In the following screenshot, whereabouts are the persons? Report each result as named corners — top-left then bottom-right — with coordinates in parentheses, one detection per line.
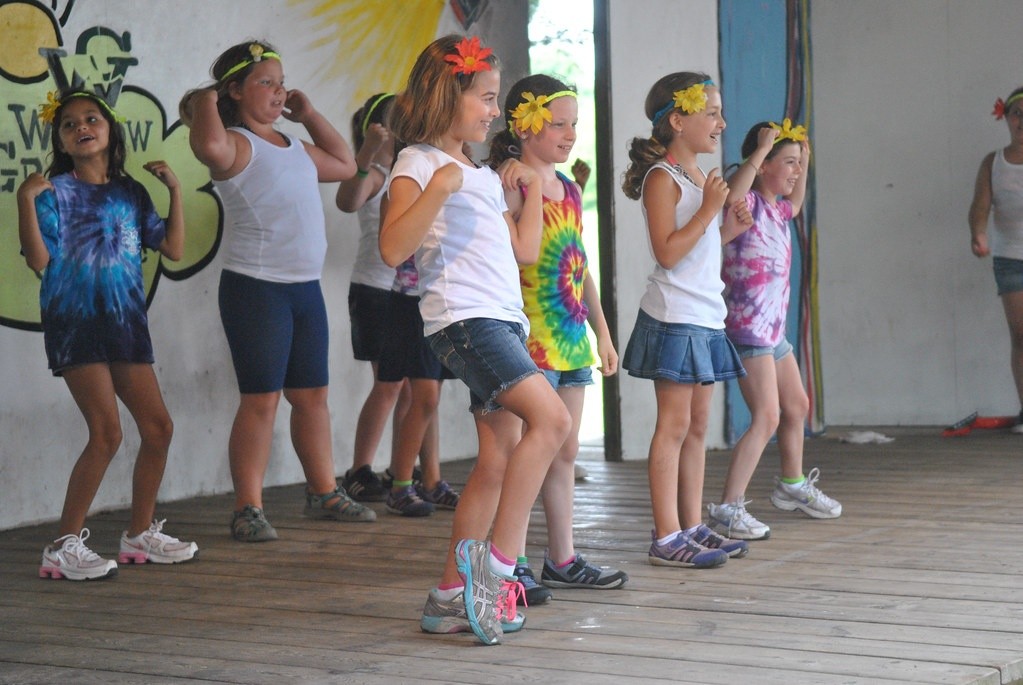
top-left (489, 73), bottom-right (631, 605)
top-left (17, 90), bottom-right (197, 579)
top-left (623, 71), bottom-right (747, 568)
top-left (335, 91), bottom-right (460, 518)
top-left (179, 41), bottom-right (377, 541)
top-left (969, 88), bottom-right (1023, 432)
top-left (707, 118), bottom-right (842, 540)
top-left (380, 34), bottom-right (571, 644)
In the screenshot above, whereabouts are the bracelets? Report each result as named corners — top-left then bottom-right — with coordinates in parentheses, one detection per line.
top-left (749, 161), bottom-right (759, 175)
top-left (356, 171), bottom-right (368, 178)
top-left (693, 214), bottom-right (706, 233)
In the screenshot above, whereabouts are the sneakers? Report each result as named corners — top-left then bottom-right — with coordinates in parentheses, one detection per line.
top-left (381, 468), bottom-right (424, 492)
top-left (540, 548), bottom-right (628, 589)
top-left (422, 481), bottom-right (459, 509)
top-left (343, 465), bottom-right (387, 502)
top-left (688, 525), bottom-right (749, 558)
top-left (648, 528), bottom-right (730, 567)
top-left (455, 538), bottom-right (527, 645)
top-left (39, 528), bottom-right (117, 581)
top-left (512, 565), bottom-right (552, 604)
top-left (118, 518), bottom-right (198, 564)
top-left (420, 587), bottom-right (526, 634)
top-left (706, 497), bottom-right (770, 540)
top-left (231, 505), bottom-right (277, 540)
top-left (304, 486), bottom-right (375, 521)
top-left (769, 467), bottom-right (842, 519)
top-left (384, 487), bottom-right (430, 517)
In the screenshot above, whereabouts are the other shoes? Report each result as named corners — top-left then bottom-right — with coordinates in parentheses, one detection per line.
top-left (574, 463), bottom-right (588, 477)
top-left (1008, 410), bottom-right (1023, 434)
